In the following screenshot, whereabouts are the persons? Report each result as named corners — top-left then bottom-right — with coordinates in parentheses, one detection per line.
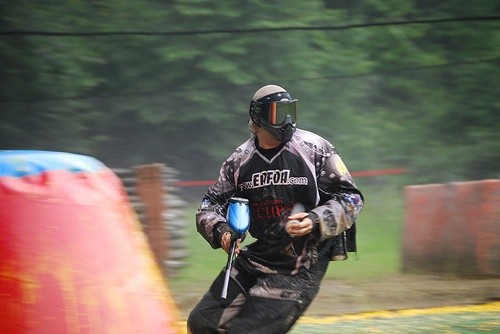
top-left (186, 82), bottom-right (366, 333)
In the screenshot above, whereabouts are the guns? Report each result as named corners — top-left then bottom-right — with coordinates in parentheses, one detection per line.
top-left (217, 196), bottom-right (251, 300)
